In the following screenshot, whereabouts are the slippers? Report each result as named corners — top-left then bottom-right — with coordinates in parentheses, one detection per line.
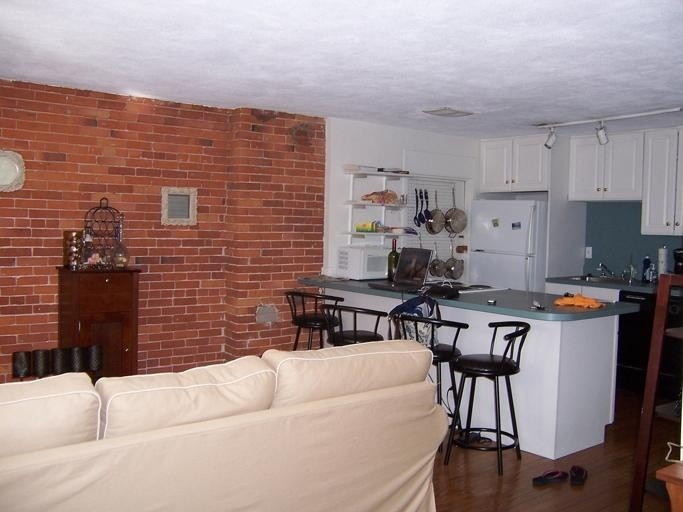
top-left (569, 465), bottom-right (587, 485)
top-left (533, 469), bottom-right (569, 487)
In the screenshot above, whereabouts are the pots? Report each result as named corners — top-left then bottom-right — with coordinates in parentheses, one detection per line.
top-left (429, 241), bottom-right (446, 277)
top-left (425, 189), bottom-right (445, 235)
top-left (444, 186), bottom-right (467, 238)
top-left (444, 240), bottom-right (463, 280)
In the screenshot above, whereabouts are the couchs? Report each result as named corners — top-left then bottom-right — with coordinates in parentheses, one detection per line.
top-left (0, 339), bottom-right (451, 512)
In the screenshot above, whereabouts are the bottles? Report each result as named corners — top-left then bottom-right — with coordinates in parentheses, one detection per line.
top-left (388, 239), bottom-right (399, 281)
top-left (656, 246), bottom-right (669, 281)
top-left (641, 255), bottom-right (650, 284)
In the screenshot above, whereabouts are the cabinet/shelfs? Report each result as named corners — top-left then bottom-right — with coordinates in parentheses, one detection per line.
top-left (480, 135), bottom-right (551, 197)
top-left (52, 266), bottom-right (142, 385)
top-left (340, 166), bottom-right (412, 246)
top-left (627, 273), bottom-right (681, 512)
top-left (638, 129), bottom-right (682, 237)
top-left (569, 133), bottom-right (644, 205)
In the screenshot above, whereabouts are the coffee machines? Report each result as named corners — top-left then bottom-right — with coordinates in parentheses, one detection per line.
top-left (672, 248), bottom-right (683, 274)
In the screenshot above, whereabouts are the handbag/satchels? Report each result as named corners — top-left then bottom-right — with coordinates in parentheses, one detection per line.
top-left (388, 297), bottom-right (440, 347)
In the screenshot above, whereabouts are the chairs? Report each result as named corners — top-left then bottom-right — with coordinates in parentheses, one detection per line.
top-left (320, 302), bottom-right (388, 346)
top-left (386, 309), bottom-right (469, 450)
top-left (442, 321), bottom-right (531, 476)
top-left (283, 290), bottom-right (344, 351)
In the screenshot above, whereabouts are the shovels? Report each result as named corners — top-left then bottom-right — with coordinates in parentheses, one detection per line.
top-left (424, 189), bottom-right (435, 223)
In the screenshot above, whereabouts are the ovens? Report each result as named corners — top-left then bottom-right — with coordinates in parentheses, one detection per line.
top-left (618, 290), bottom-right (682, 379)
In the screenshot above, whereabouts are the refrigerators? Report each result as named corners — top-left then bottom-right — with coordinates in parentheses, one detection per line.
top-left (466, 200), bottom-right (547, 294)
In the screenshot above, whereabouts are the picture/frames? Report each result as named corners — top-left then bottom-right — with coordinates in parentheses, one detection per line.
top-left (160, 187), bottom-right (198, 225)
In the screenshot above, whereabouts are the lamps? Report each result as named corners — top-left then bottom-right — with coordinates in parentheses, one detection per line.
top-left (545, 128), bottom-right (559, 150)
top-left (594, 121), bottom-right (609, 146)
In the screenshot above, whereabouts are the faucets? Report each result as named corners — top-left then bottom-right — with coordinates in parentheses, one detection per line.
top-left (596, 262), bottom-right (614, 278)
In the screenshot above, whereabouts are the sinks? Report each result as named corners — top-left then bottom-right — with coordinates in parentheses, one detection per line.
top-left (559, 275), bottom-right (608, 283)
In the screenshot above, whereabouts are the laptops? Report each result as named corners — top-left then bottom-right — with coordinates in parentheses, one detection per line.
top-left (368, 246), bottom-right (434, 292)
